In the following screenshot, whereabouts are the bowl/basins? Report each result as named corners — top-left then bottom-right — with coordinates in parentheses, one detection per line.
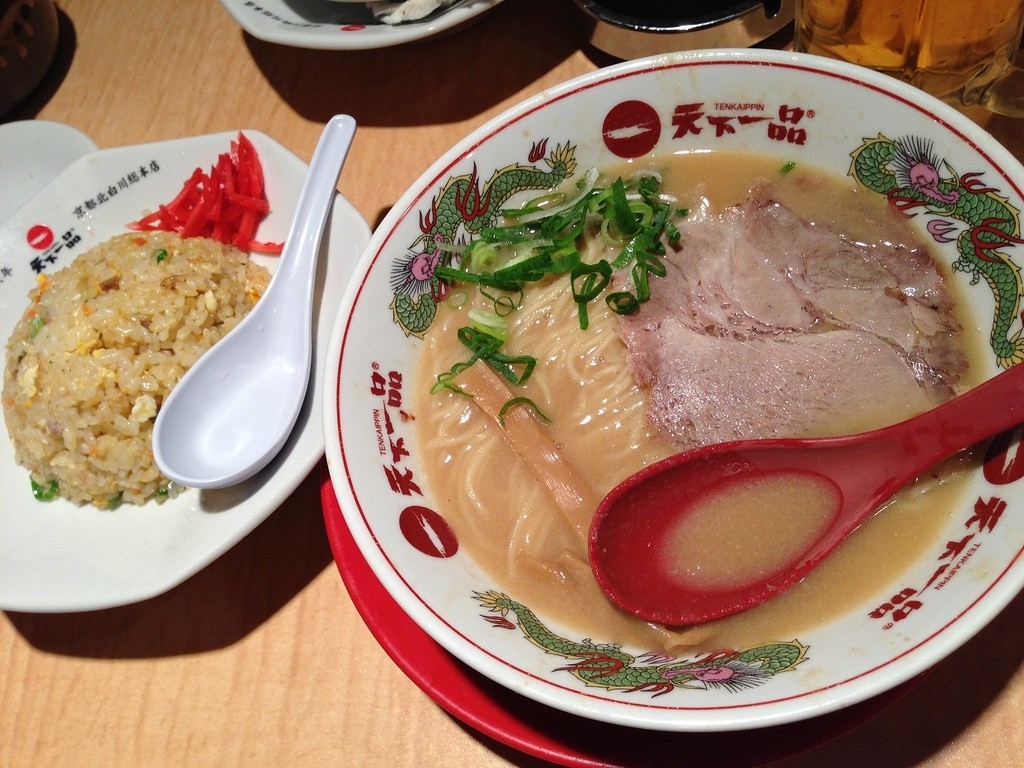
top-left (321, 48), bottom-right (1023, 735)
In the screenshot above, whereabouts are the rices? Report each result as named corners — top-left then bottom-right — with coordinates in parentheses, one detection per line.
top-left (0, 232), bottom-right (278, 508)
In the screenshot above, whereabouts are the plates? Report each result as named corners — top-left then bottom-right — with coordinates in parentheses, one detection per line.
top-left (575, 1), bottom-right (782, 34)
top-left (315, 462), bottom-right (986, 768)
top-left (1, 130), bottom-right (373, 615)
top-left (222, 1), bottom-right (504, 50)
top-left (2, 120), bottom-right (103, 228)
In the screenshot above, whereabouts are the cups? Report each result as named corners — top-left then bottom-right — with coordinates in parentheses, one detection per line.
top-left (1, 0), bottom-right (60, 121)
top-left (793, 1), bottom-right (1024, 120)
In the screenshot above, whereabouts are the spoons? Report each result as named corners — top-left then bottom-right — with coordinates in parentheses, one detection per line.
top-left (589, 357), bottom-right (1024, 627)
top-left (153, 112), bottom-right (359, 488)
